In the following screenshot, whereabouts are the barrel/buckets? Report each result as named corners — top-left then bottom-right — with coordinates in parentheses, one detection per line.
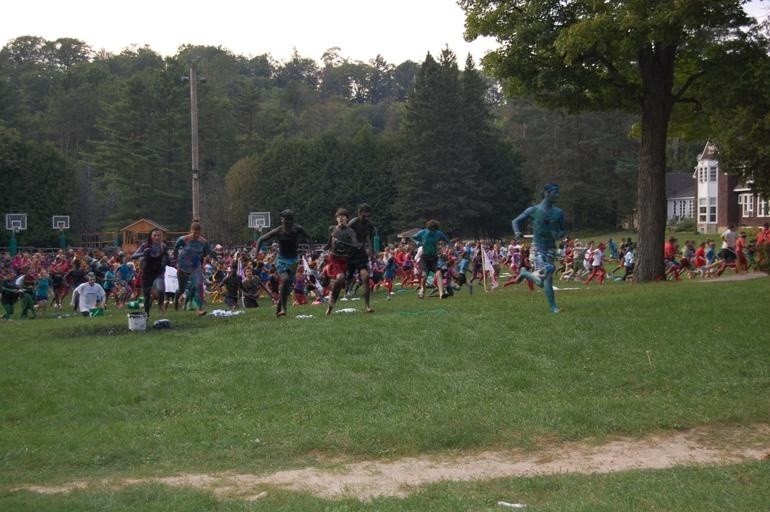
top-left (89, 308), bottom-right (104, 317)
top-left (125, 300), bottom-right (140, 310)
top-left (126, 310), bottom-right (149, 332)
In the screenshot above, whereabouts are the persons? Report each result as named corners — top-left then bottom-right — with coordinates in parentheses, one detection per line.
top-left (505, 183), bottom-right (565, 313)
top-left (254, 208), bottom-right (311, 316)
top-left (410, 219), bottom-right (452, 300)
top-left (346, 203), bottom-right (376, 314)
top-left (322, 208), bottom-right (362, 315)
top-left (0, 225), bottom-right (763, 322)
top-left (174, 222), bottom-right (212, 316)
